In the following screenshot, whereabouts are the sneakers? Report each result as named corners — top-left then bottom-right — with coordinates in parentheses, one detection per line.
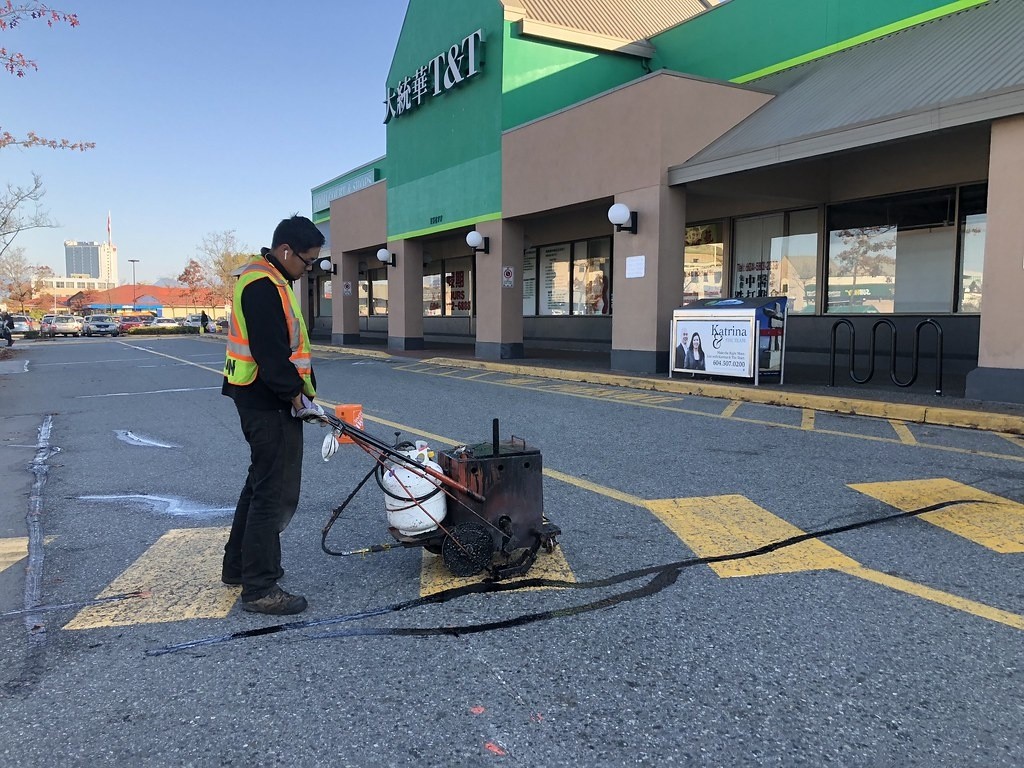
top-left (243, 587), bottom-right (307, 615)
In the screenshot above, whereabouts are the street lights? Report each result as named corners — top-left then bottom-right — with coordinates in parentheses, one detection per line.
top-left (129, 260), bottom-right (140, 311)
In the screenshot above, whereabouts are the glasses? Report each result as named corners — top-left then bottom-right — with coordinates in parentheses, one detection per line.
top-left (290, 245), bottom-right (319, 268)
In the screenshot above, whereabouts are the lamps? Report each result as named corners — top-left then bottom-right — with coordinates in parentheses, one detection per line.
top-left (320, 260), bottom-right (337, 275)
top-left (466, 231), bottom-right (489, 255)
top-left (608, 203), bottom-right (637, 234)
top-left (377, 249), bottom-right (396, 267)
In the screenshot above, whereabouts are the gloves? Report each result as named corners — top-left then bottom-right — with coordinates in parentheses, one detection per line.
top-left (291, 392), bottom-right (327, 427)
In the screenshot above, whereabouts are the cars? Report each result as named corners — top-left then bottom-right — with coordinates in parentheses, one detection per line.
top-left (47, 315), bottom-right (80, 338)
top-left (85, 314), bottom-right (118, 337)
top-left (216, 316), bottom-right (229, 333)
top-left (9, 312), bottom-right (186, 334)
top-left (182, 313), bottom-right (216, 333)
top-left (149, 317), bottom-right (179, 329)
top-left (116, 315), bottom-right (145, 337)
top-left (8, 316), bottom-right (33, 338)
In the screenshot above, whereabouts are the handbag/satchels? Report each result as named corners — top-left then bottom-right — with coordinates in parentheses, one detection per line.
top-left (200, 325), bottom-right (204, 334)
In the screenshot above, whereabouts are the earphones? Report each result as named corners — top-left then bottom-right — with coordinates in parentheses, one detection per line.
top-left (284, 250), bottom-right (288, 260)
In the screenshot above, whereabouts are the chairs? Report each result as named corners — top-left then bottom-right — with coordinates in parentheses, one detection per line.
top-left (67, 318), bottom-right (71, 322)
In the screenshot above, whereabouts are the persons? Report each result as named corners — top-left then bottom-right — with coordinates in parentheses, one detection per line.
top-left (2, 310), bottom-right (15, 347)
top-left (676, 328), bottom-right (705, 370)
top-left (201, 311), bottom-right (209, 333)
top-left (222, 212), bottom-right (329, 614)
